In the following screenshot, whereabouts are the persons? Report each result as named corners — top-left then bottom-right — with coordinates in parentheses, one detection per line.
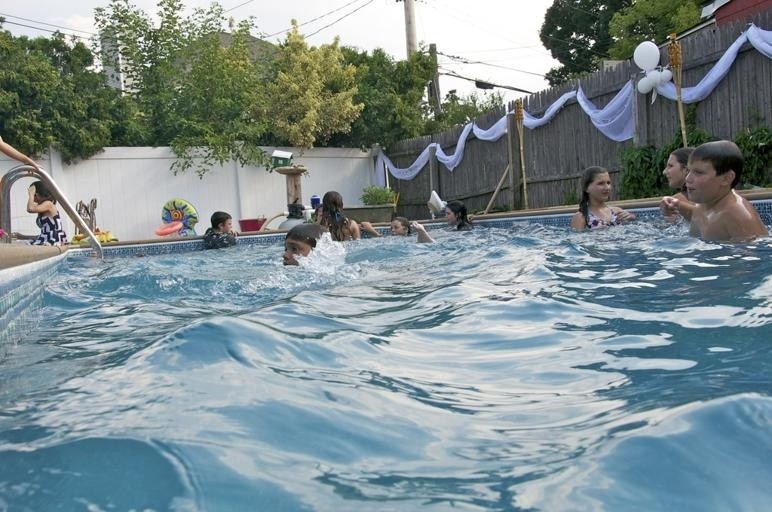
top-left (9, 180), bottom-right (68, 248)
top-left (204, 188), bottom-right (475, 270)
top-left (569, 138), bottom-right (768, 245)
top-left (0, 137), bottom-right (43, 175)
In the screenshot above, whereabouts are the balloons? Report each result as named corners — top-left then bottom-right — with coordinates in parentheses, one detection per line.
top-left (634, 40), bottom-right (674, 94)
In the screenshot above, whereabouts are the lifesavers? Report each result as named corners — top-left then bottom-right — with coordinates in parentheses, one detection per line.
top-left (162, 198), bottom-right (198, 237)
top-left (71, 231), bottom-right (119, 248)
top-left (155, 221), bottom-right (183, 236)
